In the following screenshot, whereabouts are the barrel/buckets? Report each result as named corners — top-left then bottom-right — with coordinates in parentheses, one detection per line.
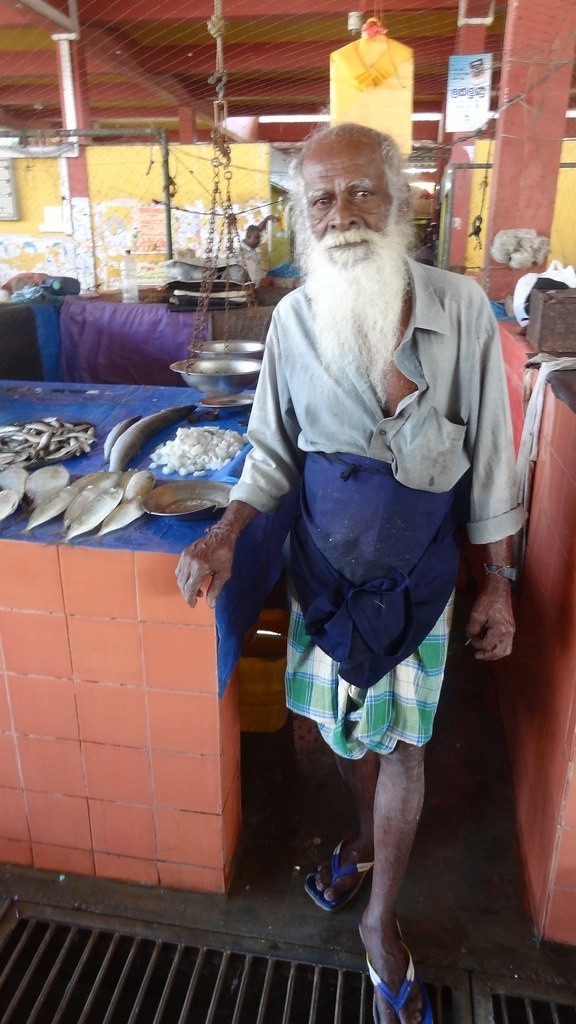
top-left (39, 275), bottom-right (81, 295)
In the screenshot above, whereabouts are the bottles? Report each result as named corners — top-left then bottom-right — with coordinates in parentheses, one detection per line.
top-left (119, 249), bottom-right (138, 303)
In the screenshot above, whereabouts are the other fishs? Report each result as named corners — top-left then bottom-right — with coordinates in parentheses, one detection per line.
top-left (0, 404), bottom-right (197, 547)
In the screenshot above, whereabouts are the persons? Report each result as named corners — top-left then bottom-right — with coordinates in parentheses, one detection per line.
top-left (175, 124), bottom-right (527, 1024)
top-left (236, 215), bottom-right (281, 288)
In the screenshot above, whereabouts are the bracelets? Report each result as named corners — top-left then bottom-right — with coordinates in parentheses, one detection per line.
top-left (482, 565), bottom-right (518, 583)
top-left (209, 525), bottom-right (240, 537)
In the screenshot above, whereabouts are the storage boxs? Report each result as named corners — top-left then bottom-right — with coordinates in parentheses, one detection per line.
top-left (239, 608), bottom-right (289, 733)
top-left (526, 289), bottom-right (576, 353)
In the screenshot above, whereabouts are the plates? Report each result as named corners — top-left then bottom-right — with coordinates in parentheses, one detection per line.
top-left (141, 481), bottom-right (237, 517)
top-left (199, 394), bottom-right (254, 408)
